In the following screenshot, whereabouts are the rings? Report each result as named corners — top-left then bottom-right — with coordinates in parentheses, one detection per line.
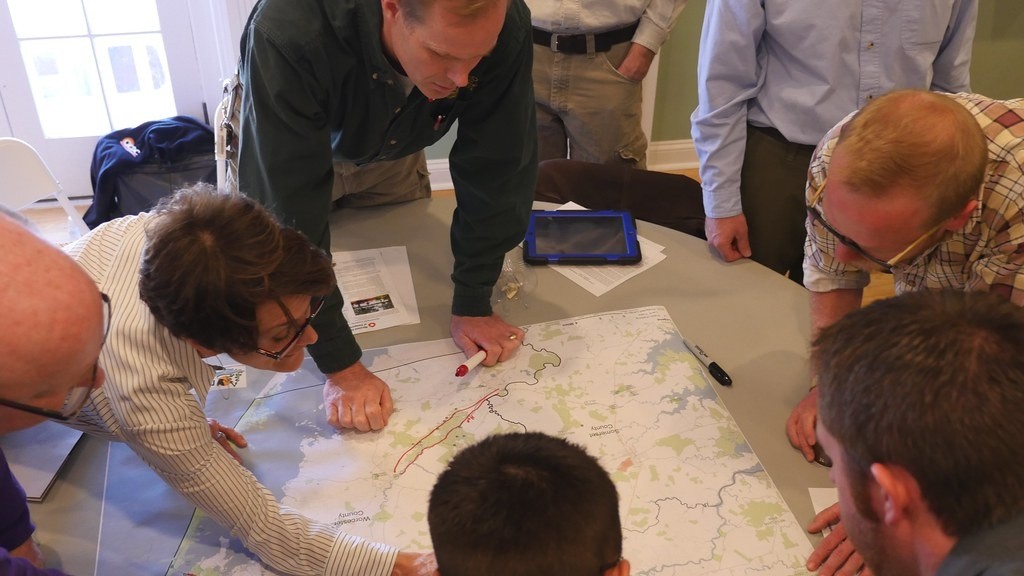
top-left (213, 431), bottom-right (224, 439)
top-left (509, 333), bottom-right (517, 340)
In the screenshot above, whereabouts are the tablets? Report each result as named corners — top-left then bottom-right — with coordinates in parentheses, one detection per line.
top-left (522, 209), bottom-right (643, 266)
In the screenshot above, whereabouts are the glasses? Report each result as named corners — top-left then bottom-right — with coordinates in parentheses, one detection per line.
top-left (806, 177), bottom-right (952, 269)
top-left (223, 294), bottom-right (325, 361)
top-left (0, 291), bottom-right (111, 423)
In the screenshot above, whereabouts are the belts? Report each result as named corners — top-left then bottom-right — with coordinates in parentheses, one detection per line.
top-left (532, 22), bottom-right (639, 55)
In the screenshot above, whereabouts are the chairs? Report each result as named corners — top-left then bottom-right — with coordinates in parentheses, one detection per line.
top-left (532, 160), bottom-right (706, 238)
top-left (0, 138), bottom-right (92, 245)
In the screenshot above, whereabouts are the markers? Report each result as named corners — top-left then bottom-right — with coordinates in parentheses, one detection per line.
top-left (454, 350), bottom-right (489, 379)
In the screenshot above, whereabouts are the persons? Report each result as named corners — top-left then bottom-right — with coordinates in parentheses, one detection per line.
top-left (50, 183), bottom-right (441, 576)
top-left (691, 0), bottom-right (979, 288)
top-left (787, 88), bottom-right (1024, 576)
top-left (214, 0), bottom-right (539, 433)
top-left (428, 431), bottom-right (631, 576)
top-left (0, 212), bottom-right (111, 576)
top-left (809, 285), bottom-right (1024, 576)
top-left (523, 0), bottom-right (690, 172)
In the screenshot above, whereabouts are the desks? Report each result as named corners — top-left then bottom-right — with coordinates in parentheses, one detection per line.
top-left (0, 196), bottom-right (852, 576)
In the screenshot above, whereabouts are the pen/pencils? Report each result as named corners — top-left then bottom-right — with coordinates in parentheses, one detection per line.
top-left (684, 338), bottom-right (732, 386)
top-left (435, 115), bottom-right (445, 132)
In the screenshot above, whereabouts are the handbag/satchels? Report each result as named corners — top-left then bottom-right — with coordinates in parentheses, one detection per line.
top-left (117, 150), bottom-right (217, 215)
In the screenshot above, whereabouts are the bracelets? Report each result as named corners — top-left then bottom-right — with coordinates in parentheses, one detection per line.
top-left (807, 374), bottom-right (821, 389)
